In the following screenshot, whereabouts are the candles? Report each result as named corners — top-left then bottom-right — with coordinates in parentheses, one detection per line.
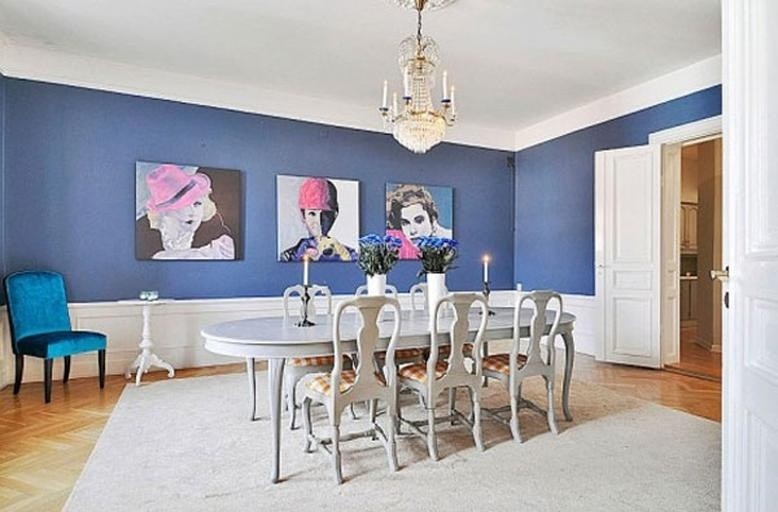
top-left (303, 255), bottom-right (309, 285)
top-left (482, 255), bottom-right (489, 282)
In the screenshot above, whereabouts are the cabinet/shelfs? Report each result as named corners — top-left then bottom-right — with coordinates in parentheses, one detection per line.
top-left (681, 204), bottom-right (698, 255)
top-left (680, 281), bottom-right (698, 320)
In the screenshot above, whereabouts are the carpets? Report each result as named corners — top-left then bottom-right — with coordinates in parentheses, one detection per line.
top-left (62, 369), bottom-right (722, 512)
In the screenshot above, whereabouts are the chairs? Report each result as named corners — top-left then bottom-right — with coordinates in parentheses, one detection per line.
top-left (411, 284), bottom-right (476, 360)
top-left (283, 284), bottom-right (358, 430)
top-left (394, 292), bottom-right (489, 462)
top-left (354, 283), bottom-right (425, 422)
top-left (3, 269), bottom-right (108, 403)
top-left (474, 290), bottom-right (564, 446)
top-left (296, 296), bottom-right (401, 482)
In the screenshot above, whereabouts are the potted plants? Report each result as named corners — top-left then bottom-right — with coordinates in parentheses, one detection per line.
top-left (356, 241), bottom-right (398, 323)
top-left (416, 244), bottom-right (458, 318)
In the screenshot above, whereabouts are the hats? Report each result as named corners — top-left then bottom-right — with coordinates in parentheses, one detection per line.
top-left (299, 178), bottom-right (338, 213)
top-left (144, 164), bottom-right (212, 213)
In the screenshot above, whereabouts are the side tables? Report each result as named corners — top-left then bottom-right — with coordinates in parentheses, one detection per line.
top-left (117, 298), bottom-right (176, 387)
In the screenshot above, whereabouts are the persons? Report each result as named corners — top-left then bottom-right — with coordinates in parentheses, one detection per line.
top-left (281, 175), bottom-right (360, 263)
top-left (385, 183), bottom-right (454, 261)
top-left (141, 163), bottom-right (235, 261)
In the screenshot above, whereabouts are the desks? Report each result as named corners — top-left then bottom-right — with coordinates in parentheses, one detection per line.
top-left (201, 306), bottom-right (576, 484)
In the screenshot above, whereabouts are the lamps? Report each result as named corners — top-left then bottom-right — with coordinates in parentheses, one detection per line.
top-left (379, 0), bottom-right (457, 156)
